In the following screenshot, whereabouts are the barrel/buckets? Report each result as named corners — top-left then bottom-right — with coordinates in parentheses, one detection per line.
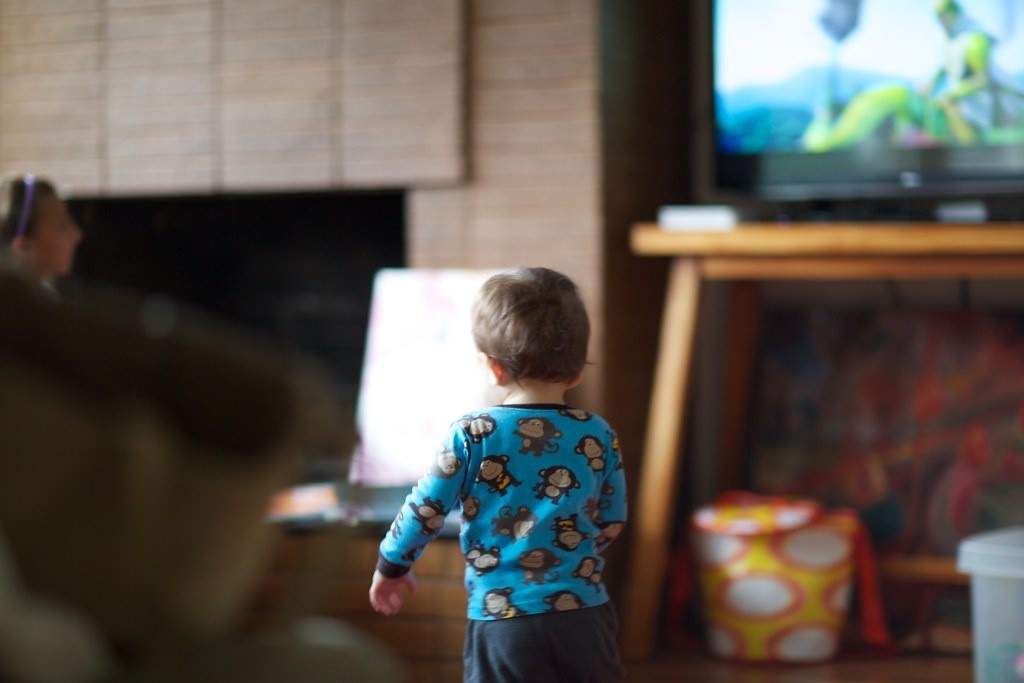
top-left (695, 491), bottom-right (857, 661)
top-left (956, 524), bottom-right (1024, 683)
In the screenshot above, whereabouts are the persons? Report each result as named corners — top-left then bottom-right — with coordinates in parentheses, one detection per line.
top-left (0, 172), bottom-right (81, 278)
top-left (367, 267), bottom-right (628, 683)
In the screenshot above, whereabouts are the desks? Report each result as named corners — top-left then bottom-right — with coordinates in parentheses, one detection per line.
top-left (612, 212), bottom-right (1023, 661)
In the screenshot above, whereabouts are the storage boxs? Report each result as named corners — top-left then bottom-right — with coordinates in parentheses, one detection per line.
top-left (956, 524), bottom-right (1023, 683)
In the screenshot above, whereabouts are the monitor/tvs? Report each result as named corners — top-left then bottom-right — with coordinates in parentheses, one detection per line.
top-left (689, 0), bottom-right (1023, 226)
